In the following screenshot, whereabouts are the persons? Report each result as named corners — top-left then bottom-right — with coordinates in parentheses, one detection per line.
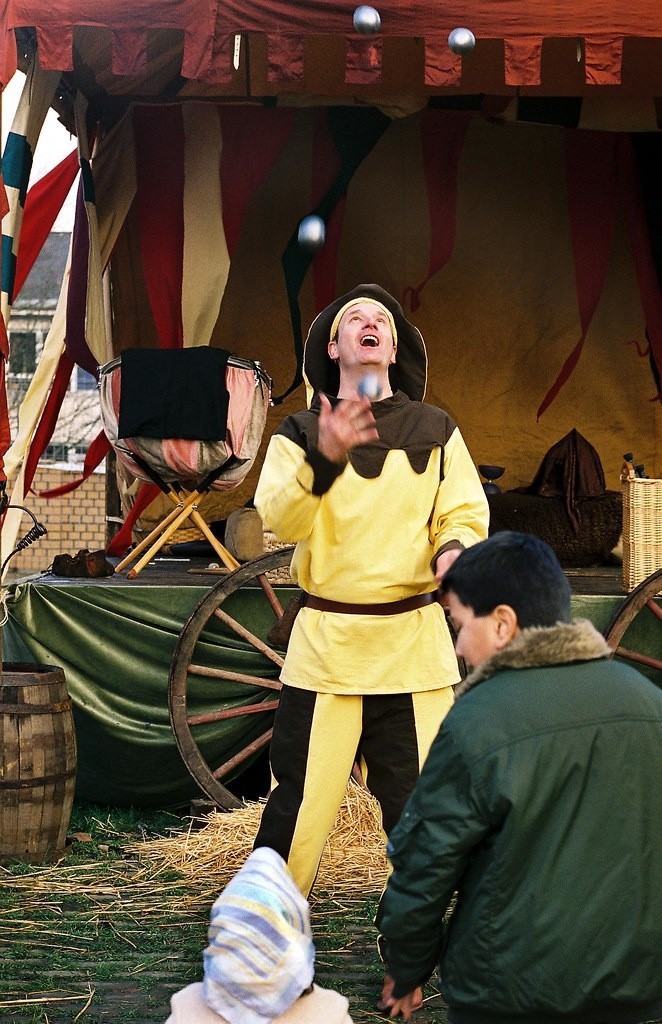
top-left (164, 847), bottom-right (352, 1024)
top-left (371, 531), bottom-right (662, 1024)
top-left (250, 285), bottom-right (490, 901)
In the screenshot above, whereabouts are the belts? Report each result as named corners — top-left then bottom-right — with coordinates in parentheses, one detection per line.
top-left (300, 590), bottom-right (440, 615)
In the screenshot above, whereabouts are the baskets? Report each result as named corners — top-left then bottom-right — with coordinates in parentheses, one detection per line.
top-left (142, 521), bottom-right (208, 556)
top-left (620, 460), bottom-right (662, 597)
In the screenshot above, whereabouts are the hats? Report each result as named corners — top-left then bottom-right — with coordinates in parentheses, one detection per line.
top-left (302, 283), bottom-right (428, 413)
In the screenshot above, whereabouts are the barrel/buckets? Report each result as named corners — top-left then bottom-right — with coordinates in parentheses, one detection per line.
top-left (0, 662), bottom-right (77, 866)
